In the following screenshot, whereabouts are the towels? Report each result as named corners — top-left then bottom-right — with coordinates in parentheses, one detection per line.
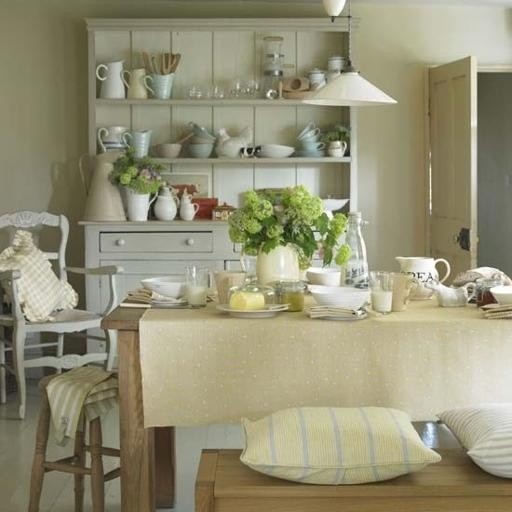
top-left (125, 286), bottom-right (188, 305)
top-left (46, 365), bottom-right (118, 448)
top-left (306, 305), bottom-right (369, 320)
top-left (481, 304), bottom-right (511, 320)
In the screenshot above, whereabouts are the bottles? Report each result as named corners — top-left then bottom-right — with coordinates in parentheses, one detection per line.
top-left (342, 212), bottom-right (368, 288)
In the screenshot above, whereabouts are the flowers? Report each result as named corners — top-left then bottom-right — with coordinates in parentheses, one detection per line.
top-left (109, 147), bottom-right (168, 196)
top-left (323, 123), bottom-right (351, 142)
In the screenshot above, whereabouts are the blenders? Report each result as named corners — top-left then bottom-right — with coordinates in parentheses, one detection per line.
top-left (262, 35), bottom-right (286, 100)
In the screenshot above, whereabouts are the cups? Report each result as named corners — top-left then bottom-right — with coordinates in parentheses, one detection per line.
top-left (475, 279), bottom-right (504, 307)
top-left (184, 265), bottom-right (208, 308)
top-left (370, 271), bottom-right (420, 313)
top-left (186, 79), bottom-right (261, 99)
top-left (297, 122), bottom-right (346, 156)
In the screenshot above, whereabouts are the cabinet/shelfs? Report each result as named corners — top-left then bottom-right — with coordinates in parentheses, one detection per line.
top-left (84, 17), bottom-right (360, 225)
top-left (76, 220), bottom-right (346, 372)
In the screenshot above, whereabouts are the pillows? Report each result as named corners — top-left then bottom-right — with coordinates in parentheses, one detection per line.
top-left (239, 406), bottom-right (441, 486)
top-left (0, 229), bottom-right (79, 322)
top-left (436, 408), bottom-right (511, 479)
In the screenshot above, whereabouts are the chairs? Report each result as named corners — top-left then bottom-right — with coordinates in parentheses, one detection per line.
top-left (2, 210), bottom-right (125, 419)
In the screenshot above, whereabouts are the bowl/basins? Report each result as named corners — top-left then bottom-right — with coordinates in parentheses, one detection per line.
top-left (322, 198), bottom-right (350, 210)
top-left (141, 268), bottom-right (371, 309)
top-left (156, 123), bottom-right (217, 158)
top-left (490, 285), bottom-right (512, 304)
top-left (256, 145), bottom-right (295, 157)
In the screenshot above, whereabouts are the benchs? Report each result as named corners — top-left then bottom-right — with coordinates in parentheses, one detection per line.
top-left (193, 447), bottom-right (512, 512)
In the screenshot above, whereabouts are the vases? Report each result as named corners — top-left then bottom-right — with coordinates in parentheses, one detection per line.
top-left (122, 185), bottom-right (150, 221)
top-left (225, 187), bottom-right (349, 269)
top-left (256, 241), bottom-right (300, 289)
top-left (328, 140), bottom-right (347, 157)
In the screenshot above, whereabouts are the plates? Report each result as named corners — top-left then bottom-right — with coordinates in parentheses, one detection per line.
top-left (216, 304), bottom-right (288, 317)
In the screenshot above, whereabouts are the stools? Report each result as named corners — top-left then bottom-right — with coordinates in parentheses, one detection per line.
top-left (29, 375), bottom-right (119, 511)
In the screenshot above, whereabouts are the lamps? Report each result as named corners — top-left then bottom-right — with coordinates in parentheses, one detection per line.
top-left (299, 0), bottom-right (398, 107)
top-left (323, 0), bottom-right (346, 23)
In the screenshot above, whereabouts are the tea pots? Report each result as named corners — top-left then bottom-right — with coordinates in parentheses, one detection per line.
top-left (153, 180), bottom-right (199, 220)
